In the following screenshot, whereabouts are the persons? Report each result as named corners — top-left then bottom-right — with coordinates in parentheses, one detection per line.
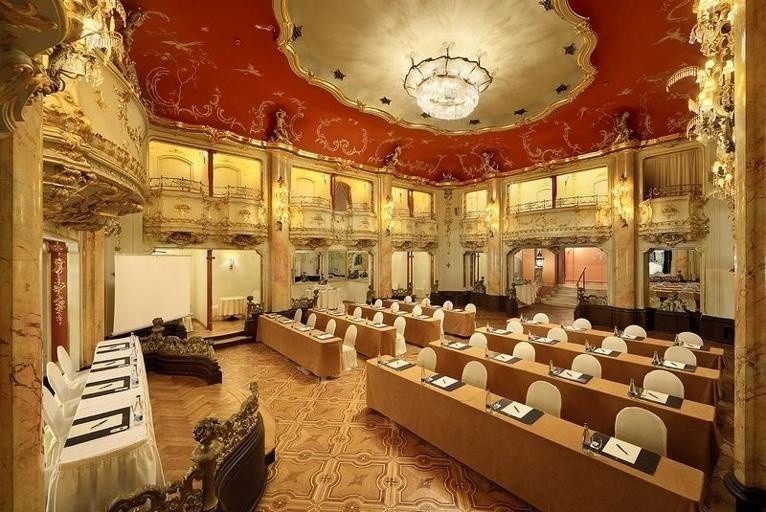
top-left (274, 110), bottom-right (286, 138)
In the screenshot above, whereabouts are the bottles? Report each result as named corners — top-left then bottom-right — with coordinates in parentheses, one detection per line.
top-left (485, 386), bottom-right (492, 410)
top-left (377, 352), bottom-right (381, 365)
top-left (129, 330), bottom-right (144, 426)
top-left (581, 423), bottom-right (591, 454)
top-left (440, 319), bottom-right (682, 374)
top-left (627, 377), bottom-right (638, 397)
top-left (261, 295), bottom-right (467, 334)
top-left (421, 366), bottom-right (426, 382)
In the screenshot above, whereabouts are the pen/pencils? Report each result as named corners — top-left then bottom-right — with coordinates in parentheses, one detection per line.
top-left (616, 445), bottom-right (627, 455)
top-left (109, 346), bottom-right (117, 349)
top-left (454, 343), bottom-right (458, 347)
top-left (501, 356), bottom-right (506, 359)
top-left (90, 419), bottom-right (108, 429)
top-left (105, 362), bottom-right (115, 365)
top-left (670, 362), bottom-right (677, 366)
top-left (513, 406), bottom-right (519, 413)
top-left (600, 348), bottom-right (605, 352)
top-left (566, 372), bottom-right (573, 377)
top-left (687, 344), bottom-right (693, 347)
top-left (99, 384), bottom-right (113, 389)
top-left (648, 393), bottom-right (659, 399)
top-left (396, 361), bottom-right (398, 366)
top-left (543, 339), bottom-right (546, 341)
top-left (626, 335), bottom-right (631, 337)
top-left (443, 379), bottom-right (446, 385)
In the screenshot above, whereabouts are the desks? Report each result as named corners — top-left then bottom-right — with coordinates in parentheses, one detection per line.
top-left (427, 336), bottom-right (725, 479)
top-left (218, 296), bottom-right (247, 322)
top-left (306, 307), bottom-right (397, 358)
top-left (257, 313), bottom-right (344, 380)
top-left (365, 354), bottom-right (706, 512)
top-left (347, 302), bottom-right (442, 349)
top-left (473, 324), bottom-right (720, 416)
top-left (507, 317), bottom-right (726, 376)
top-left (43, 330), bottom-right (165, 511)
top-left (383, 297), bottom-right (476, 340)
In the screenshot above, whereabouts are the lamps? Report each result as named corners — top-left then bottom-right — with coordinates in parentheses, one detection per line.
top-left (483, 196), bottom-right (499, 242)
top-left (400, 40), bottom-right (496, 123)
top-left (37, 2), bottom-right (129, 95)
top-left (609, 173), bottom-right (633, 229)
top-left (533, 250), bottom-right (547, 268)
top-left (272, 172), bottom-right (290, 232)
top-left (665, 1), bottom-right (739, 230)
top-left (382, 193), bottom-right (395, 238)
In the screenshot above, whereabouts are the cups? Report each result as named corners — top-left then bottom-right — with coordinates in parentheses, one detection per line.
top-left (591, 437), bottom-right (603, 456)
top-left (637, 388), bottom-right (648, 398)
top-left (491, 398), bottom-right (501, 409)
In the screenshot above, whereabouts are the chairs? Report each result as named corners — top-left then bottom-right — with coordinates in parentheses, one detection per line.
top-left (469, 332), bottom-right (488, 350)
top-left (663, 346), bottom-right (697, 366)
top-left (532, 312), bottom-right (550, 324)
top-left (675, 332), bottom-right (704, 345)
top-left (461, 360), bottom-right (487, 391)
top-left (572, 318), bottom-right (592, 331)
top-left (36, 382), bottom-right (71, 444)
top-left (613, 406), bottom-right (668, 458)
top-left (512, 342), bottom-right (536, 363)
top-left (247, 289), bottom-right (263, 304)
top-left (373, 299), bottom-right (446, 340)
top-left (403, 295), bottom-right (477, 332)
top-left (547, 328), bottom-right (568, 341)
top-left (337, 302), bottom-right (407, 357)
top-left (641, 369), bottom-right (686, 398)
top-left (506, 321), bottom-right (524, 333)
top-left (54, 343), bottom-right (91, 392)
top-left (45, 361), bottom-right (85, 417)
top-left (415, 346), bottom-right (437, 371)
top-left (623, 324), bottom-right (647, 338)
top-left (601, 336), bottom-right (628, 353)
top-left (293, 308), bottom-right (359, 371)
top-left (524, 379), bottom-right (563, 419)
top-left (570, 353), bottom-right (602, 380)
